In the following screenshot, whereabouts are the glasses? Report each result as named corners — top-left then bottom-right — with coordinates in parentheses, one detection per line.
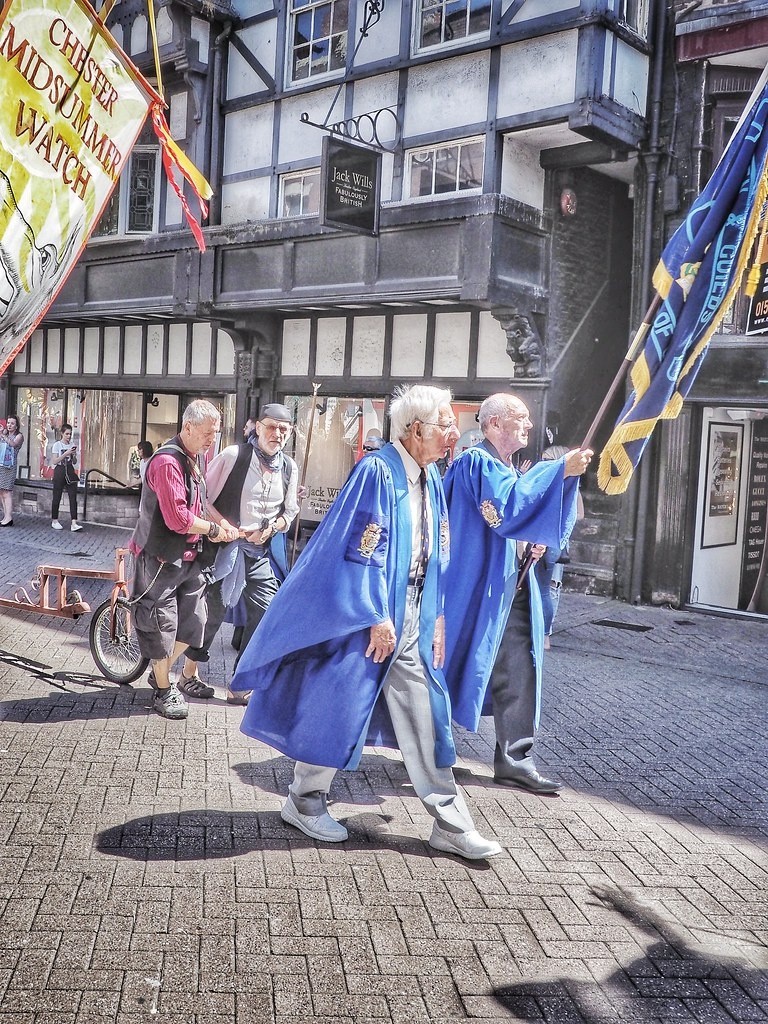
top-left (406, 418), bottom-right (457, 430)
top-left (259, 420), bottom-right (291, 434)
top-left (363, 445), bottom-right (380, 451)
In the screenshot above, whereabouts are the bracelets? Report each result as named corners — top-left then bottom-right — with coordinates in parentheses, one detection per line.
top-left (208, 522), bottom-right (219, 538)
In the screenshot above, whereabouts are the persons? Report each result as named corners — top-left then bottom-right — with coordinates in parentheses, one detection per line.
top-left (126, 441), bottom-right (153, 516)
top-left (127, 399), bottom-right (227, 719)
top-left (51, 423), bottom-right (83, 531)
top-left (363, 436), bottom-right (386, 455)
top-left (281, 385), bottom-right (502, 859)
top-left (205, 403), bottom-right (300, 676)
top-left (442, 393), bottom-right (594, 792)
top-left (520, 446), bottom-right (585, 649)
top-left (243, 416), bottom-right (259, 443)
top-left (0, 414), bottom-right (24, 527)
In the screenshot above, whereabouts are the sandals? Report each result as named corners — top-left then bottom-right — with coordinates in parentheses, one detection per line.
top-left (177, 673), bottom-right (214, 698)
top-left (226, 683), bottom-right (252, 705)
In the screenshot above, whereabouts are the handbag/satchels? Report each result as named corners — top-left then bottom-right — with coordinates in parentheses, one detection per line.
top-left (0, 437), bottom-right (14, 468)
top-left (65, 473), bottom-right (80, 485)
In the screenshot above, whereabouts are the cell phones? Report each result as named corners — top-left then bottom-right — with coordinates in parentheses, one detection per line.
top-left (71, 446), bottom-right (77, 451)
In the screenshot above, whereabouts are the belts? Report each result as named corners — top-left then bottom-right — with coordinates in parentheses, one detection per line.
top-left (408, 577), bottom-right (425, 587)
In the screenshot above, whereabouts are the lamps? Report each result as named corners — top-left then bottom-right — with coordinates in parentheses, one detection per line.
top-left (51, 393), bottom-right (57, 401)
top-left (152, 398), bottom-right (159, 407)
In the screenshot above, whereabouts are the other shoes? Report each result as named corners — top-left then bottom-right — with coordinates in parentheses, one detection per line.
top-left (71, 524), bottom-right (83, 531)
top-left (52, 522), bottom-right (63, 530)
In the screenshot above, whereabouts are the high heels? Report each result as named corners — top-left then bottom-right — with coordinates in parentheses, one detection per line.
top-left (0, 519), bottom-right (13, 527)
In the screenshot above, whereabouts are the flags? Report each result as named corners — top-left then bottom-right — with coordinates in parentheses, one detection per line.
top-left (597, 62), bottom-right (768, 496)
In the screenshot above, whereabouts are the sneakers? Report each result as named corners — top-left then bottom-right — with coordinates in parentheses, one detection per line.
top-left (429, 819), bottom-right (502, 859)
top-left (148, 671), bottom-right (159, 689)
top-left (153, 686), bottom-right (188, 718)
top-left (281, 793), bottom-right (348, 841)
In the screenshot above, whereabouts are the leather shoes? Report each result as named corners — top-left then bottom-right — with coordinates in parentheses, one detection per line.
top-left (494, 767), bottom-right (564, 793)
